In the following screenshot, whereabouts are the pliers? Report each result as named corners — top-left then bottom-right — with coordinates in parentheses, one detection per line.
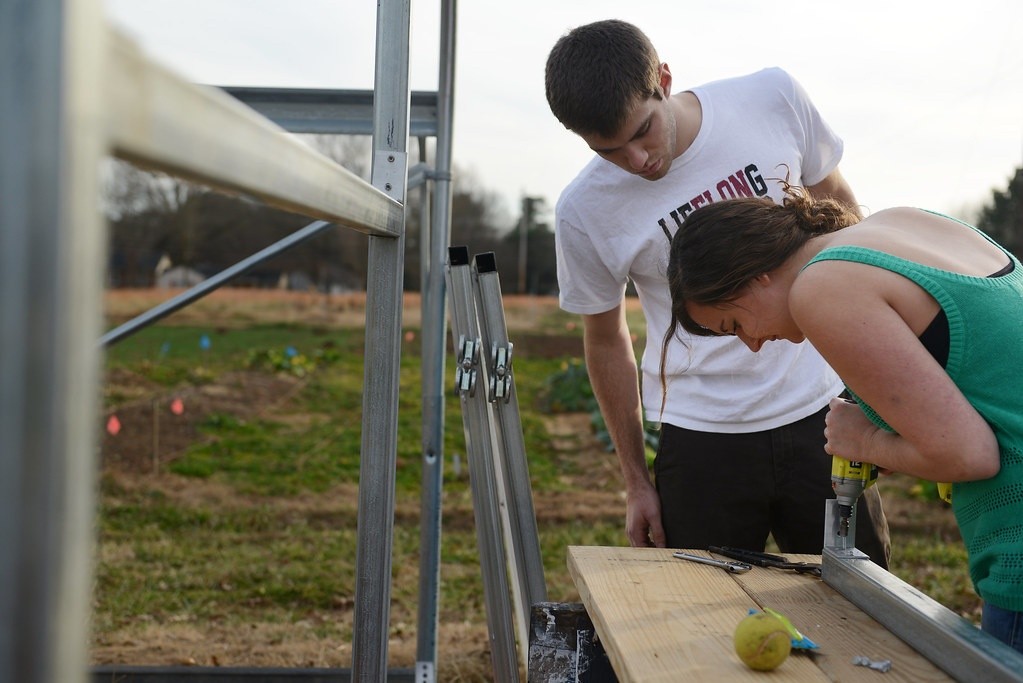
top-left (709, 545), bottom-right (823, 577)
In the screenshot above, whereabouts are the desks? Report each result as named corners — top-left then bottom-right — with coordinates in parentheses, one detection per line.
top-left (566, 545), bottom-right (956, 683)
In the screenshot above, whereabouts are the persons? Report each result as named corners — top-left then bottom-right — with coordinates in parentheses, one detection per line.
top-left (666, 192), bottom-right (1023, 659)
top-left (544, 18), bottom-right (893, 573)
